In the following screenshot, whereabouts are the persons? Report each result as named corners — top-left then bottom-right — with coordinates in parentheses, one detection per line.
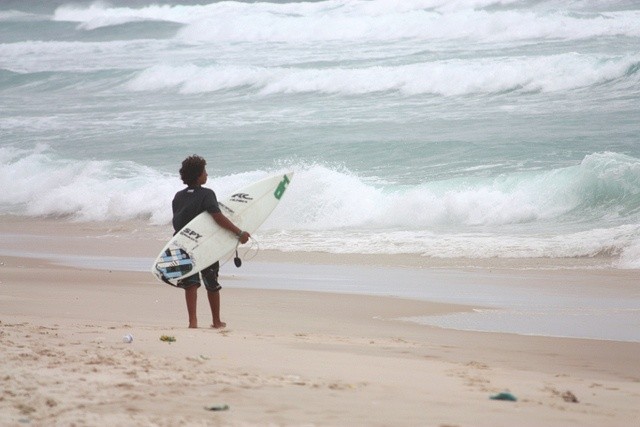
top-left (172, 152), bottom-right (251, 328)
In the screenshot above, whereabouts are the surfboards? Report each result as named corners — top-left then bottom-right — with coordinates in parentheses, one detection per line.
top-left (152, 171), bottom-right (295, 286)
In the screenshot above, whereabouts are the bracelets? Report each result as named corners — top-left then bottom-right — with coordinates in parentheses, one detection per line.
top-left (237, 230), bottom-right (243, 236)
